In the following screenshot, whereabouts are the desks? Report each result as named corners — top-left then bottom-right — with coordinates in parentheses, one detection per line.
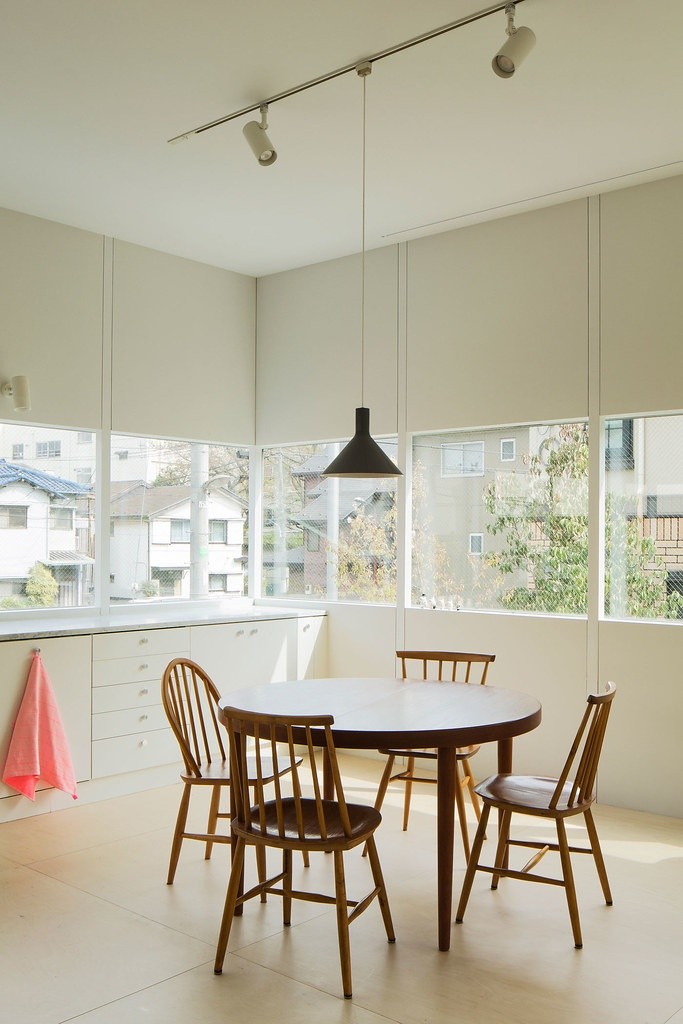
top-left (217, 678), bottom-right (541, 952)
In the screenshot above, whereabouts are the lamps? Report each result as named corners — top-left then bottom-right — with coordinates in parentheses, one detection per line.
top-left (320, 74), bottom-right (405, 477)
top-left (491, 25), bottom-right (537, 78)
top-left (243, 121), bottom-right (278, 166)
top-left (4, 374), bottom-right (29, 413)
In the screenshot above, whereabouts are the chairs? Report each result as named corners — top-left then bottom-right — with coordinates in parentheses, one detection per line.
top-left (362, 651), bottom-right (496, 867)
top-left (161, 657), bottom-right (305, 887)
top-left (454, 680), bottom-right (618, 949)
top-left (213, 707), bottom-right (399, 1001)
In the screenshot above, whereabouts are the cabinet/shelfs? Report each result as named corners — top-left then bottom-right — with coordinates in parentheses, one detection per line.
top-left (287, 613), bottom-right (328, 685)
top-left (187, 618), bottom-right (292, 761)
top-left (0, 634), bottom-right (92, 823)
top-left (91, 625), bottom-right (193, 782)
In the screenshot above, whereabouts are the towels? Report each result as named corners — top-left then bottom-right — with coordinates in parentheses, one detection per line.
top-left (1, 653), bottom-right (80, 802)
